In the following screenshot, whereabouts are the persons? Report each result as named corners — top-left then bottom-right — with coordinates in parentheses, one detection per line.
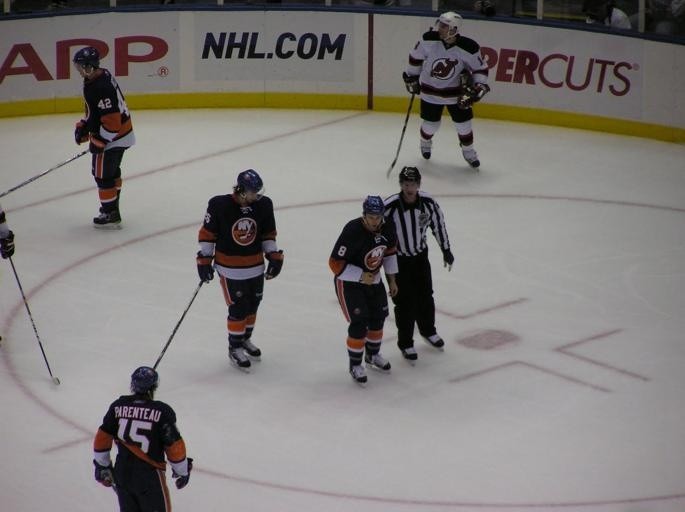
top-left (585, 3), bottom-right (632, 31)
top-left (328, 196), bottom-right (400, 385)
top-left (93, 366), bottom-right (190, 512)
top-left (197, 170), bottom-right (283, 368)
top-left (0, 209), bottom-right (15, 259)
top-left (71, 47), bottom-right (136, 231)
top-left (404, 11), bottom-right (488, 169)
top-left (383, 167), bottom-right (455, 360)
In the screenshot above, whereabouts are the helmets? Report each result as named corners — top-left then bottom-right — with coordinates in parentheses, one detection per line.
top-left (399, 166), bottom-right (423, 188)
top-left (360, 192), bottom-right (386, 232)
top-left (73, 48), bottom-right (100, 77)
top-left (236, 168), bottom-right (265, 204)
top-left (128, 366), bottom-right (160, 394)
top-left (435, 12), bottom-right (462, 42)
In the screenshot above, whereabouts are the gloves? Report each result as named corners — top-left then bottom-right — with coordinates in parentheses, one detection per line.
top-left (0, 231), bottom-right (17, 260)
top-left (402, 73), bottom-right (422, 95)
top-left (192, 253), bottom-right (215, 284)
top-left (73, 119), bottom-right (89, 146)
top-left (263, 251), bottom-right (288, 281)
top-left (466, 85), bottom-right (485, 102)
top-left (90, 458), bottom-right (115, 489)
top-left (442, 251), bottom-right (454, 273)
top-left (87, 134), bottom-right (111, 155)
top-left (170, 465), bottom-right (192, 489)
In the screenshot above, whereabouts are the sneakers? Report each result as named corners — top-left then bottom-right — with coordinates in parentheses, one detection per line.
top-left (348, 363), bottom-right (369, 388)
top-left (245, 341), bottom-right (263, 362)
top-left (226, 342), bottom-right (254, 373)
top-left (418, 326), bottom-right (446, 354)
top-left (364, 353), bottom-right (394, 375)
top-left (92, 211), bottom-right (122, 232)
top-left (396, 339), bottom-right (420, 366)
top-left (99, 200), bottom-right (119, 214)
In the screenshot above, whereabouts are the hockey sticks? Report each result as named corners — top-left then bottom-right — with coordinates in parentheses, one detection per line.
top-left (6, 252), bottom-right (63, 387)
top-left (385, 90), bottom-right (416, 180)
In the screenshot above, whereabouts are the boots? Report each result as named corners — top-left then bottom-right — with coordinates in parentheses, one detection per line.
top-left (418, 137), bottom-right (435, 159)
top-left (458, 145), bottom-right (481, 168)
